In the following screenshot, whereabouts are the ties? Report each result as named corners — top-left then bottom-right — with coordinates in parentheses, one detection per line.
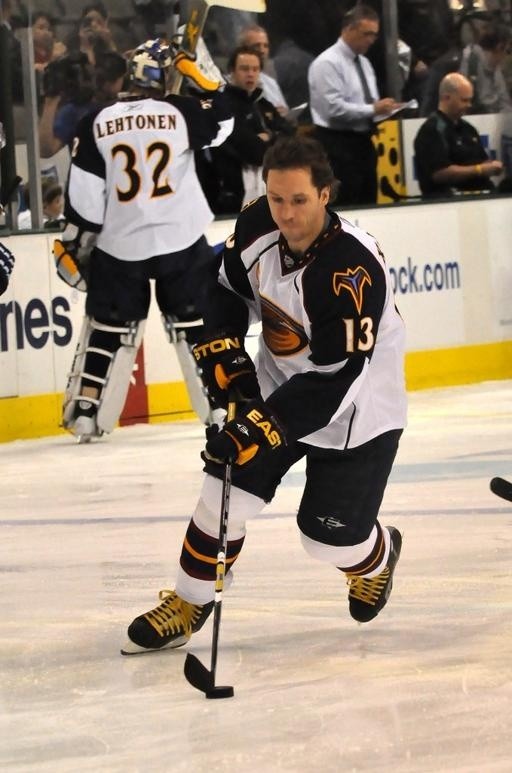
top-left (354, 57), bottom-right (375, 106)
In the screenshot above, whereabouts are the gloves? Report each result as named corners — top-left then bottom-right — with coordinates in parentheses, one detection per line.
top-left (200, 406), bottom-right (290, 466)
top-left (168, 22), bottom-right (227, 94)
top-left (53, 235), bottom-right (91, 293)
top-left (192, 336), bottom-right (265, 418)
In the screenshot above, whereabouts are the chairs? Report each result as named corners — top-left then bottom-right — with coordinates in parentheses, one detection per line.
top-left (12, 0), bottom-right (155, 145)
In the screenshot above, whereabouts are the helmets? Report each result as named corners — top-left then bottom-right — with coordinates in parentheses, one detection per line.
top-left (125, 37), bottom-right (183, 100)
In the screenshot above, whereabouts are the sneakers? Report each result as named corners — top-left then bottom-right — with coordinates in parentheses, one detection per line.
top-left (347, 526), bottom-right (402, 624)
top-left (127, 590), bottom-right (215, 651)
top-left (71, 385), bottom-right (103, 438)
top-left (207, 408), bottom-right (228, 434)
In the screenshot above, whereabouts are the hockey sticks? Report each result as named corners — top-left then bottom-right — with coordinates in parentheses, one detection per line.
top-left (490, 477), bottom-right (511, 502)
top-left (184, 400), bottom-right (238, 692)
top-left (165, 0), bottom-right (266, 95)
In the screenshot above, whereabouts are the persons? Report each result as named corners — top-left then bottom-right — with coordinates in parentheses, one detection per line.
top-left (51, 31), bottom-right (261, 447)
top-left (0, 242), bottom-right (18, 291)
top-left (128, 133), bottom-right (408, 653)
top-left (1, 0), bottom-right (512, 242)
